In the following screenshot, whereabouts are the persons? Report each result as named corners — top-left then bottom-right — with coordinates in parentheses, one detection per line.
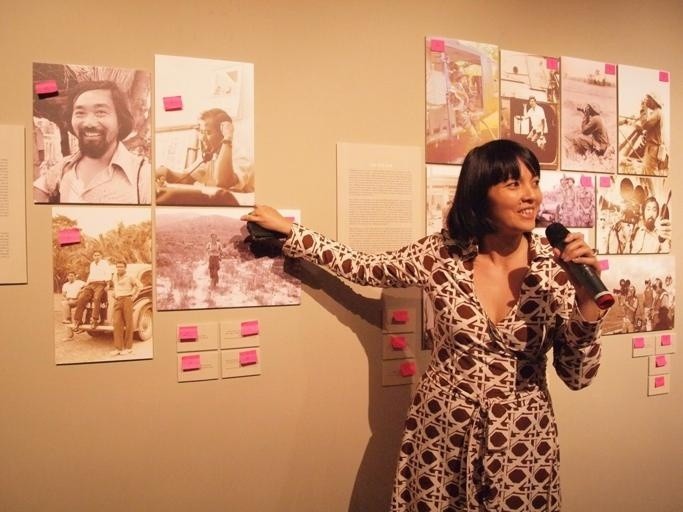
top-left (524, 97), bottom-right (548, 147)
top-left (239, 139), bottom-right (612, 512)
top-left (599, 178), bottom-right (672, 254)
top-left (613, 276), bottom-right (674, 334)
top-left (636, 92), bottom-right (666, 176)
top-left (70, 250), bottom-right (115, 330)
top-left (153, 108), bottom-right (254, 194)
top-left (109, 260), bottom-right (144, 358)
top-left (32, 80), bottom-right (152, 205)
top-left (60, 271), bottom-right (87, 342)
top-left (204, 233), bottom-right (224, 290)
top-left (556, 176), bottom-right (595, 227)
top-left (575, 103), bottom-right (609, 156)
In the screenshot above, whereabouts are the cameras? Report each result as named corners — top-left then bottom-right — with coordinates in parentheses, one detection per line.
top-left (576, 102), bottom-right (589, 113)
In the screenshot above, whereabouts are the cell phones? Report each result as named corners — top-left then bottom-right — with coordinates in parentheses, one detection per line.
top-left (246, 220), bottom-right (289, 242)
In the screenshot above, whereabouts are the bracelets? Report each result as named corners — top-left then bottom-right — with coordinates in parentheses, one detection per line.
top-left (220, 140), bottom-right (232, 144)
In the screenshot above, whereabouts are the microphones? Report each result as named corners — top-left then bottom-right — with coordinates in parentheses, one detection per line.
top-left (544, 221), bottom-right (617, 310)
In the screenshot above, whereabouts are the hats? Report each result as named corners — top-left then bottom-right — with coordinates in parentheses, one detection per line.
top-left (567, 177), bottom-right (575, 183)
top-left (647, 92), bottom-right (664, 108)
top-left (451, 71), bottom-right (468, 81)
top-left (588, 103), bottom-right (601, 115)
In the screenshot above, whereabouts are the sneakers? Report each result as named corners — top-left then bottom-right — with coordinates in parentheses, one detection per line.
top-left (63, 338), bottom-right (73, 341)
top-left (62, 320), bottom-right (71, 324)
top-left (112, 349), bottom-right (133, 356)
top-left (91, 321), bottom-right (97, 329)
top-left (75, 321), bottom-right (82, 329)
